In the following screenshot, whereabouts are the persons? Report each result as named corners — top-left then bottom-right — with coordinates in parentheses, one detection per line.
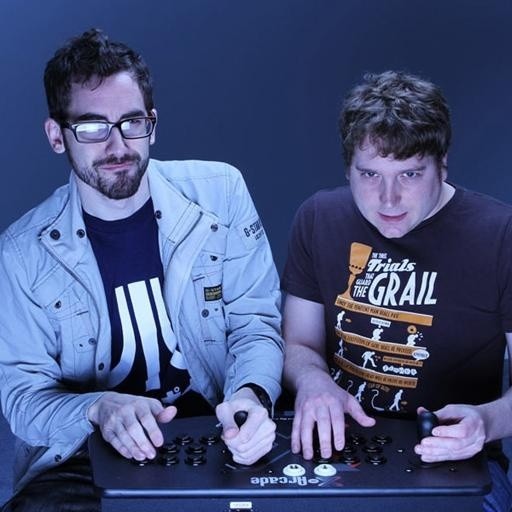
top-left (0, 27), bottom-right (286, 512)
top-left (281, 70), bottom-right (512, 512)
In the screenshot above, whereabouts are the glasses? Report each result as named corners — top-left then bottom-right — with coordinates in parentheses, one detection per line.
top-left (65, 111), bottom-right (158, 143)
